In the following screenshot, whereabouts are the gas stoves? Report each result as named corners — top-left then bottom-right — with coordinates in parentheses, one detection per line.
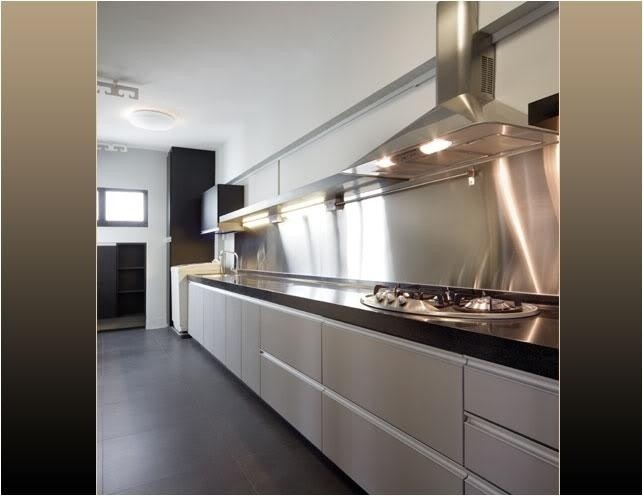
top-left (361, 281), bottom-right (540, 322)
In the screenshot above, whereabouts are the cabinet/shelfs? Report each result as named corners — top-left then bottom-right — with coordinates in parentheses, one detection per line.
top-left (225, 289), bottom-right (261, 398)
top-left (187, 280), bottom-right (226, 367)
top-left (96, 243), bottom-right (145, 319)
top-left (464, 353), bottom-right (560, 494)
top-left (260, 300), bottom-right (467, 495)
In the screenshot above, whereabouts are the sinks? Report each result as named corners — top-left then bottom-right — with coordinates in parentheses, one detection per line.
top-left (194, 272), bottom-right (232, 276)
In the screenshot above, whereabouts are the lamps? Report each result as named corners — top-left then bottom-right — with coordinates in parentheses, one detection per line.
top-left (127, 109), bottom-right (178, 132)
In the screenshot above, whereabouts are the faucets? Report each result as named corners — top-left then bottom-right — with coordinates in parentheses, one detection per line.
top-left (220, 249), bottom-right (239, 275)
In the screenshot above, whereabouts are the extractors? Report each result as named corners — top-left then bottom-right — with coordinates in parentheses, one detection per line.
top-left (340, 2), bottom-right (559, 180)
